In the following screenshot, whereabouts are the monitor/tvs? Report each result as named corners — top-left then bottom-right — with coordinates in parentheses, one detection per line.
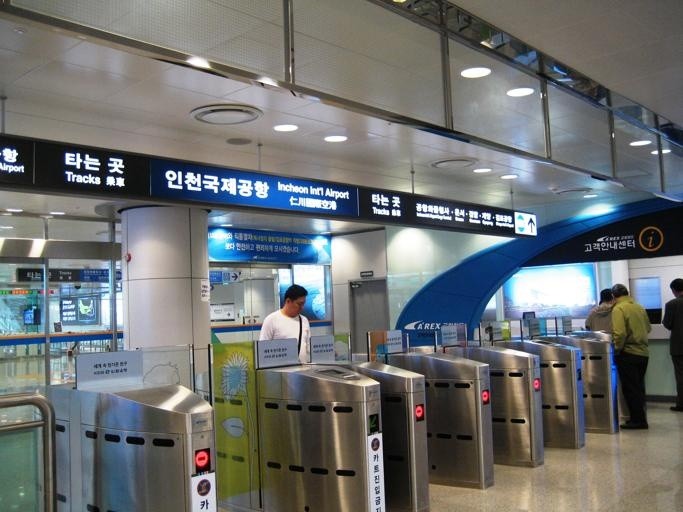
top-left (645, 308), bottom-right (662, 324)
top-left (523, 311), bottom-right (536, 327)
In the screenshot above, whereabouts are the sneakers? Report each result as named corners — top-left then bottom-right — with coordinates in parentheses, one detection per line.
top-left (671, 406), bottom-right (682, 411)
top-left (621, 419), bottom-right (648, 429)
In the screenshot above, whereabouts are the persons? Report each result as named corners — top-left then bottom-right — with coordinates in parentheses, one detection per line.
top-left (583, 288), bottom-right (614, 334)
top-left (660, 278), bottom-right (682, 412)
top-left (257, 285), bottom-right (311, 364)
top-left (608, 282), bottom-right (652, 430)
